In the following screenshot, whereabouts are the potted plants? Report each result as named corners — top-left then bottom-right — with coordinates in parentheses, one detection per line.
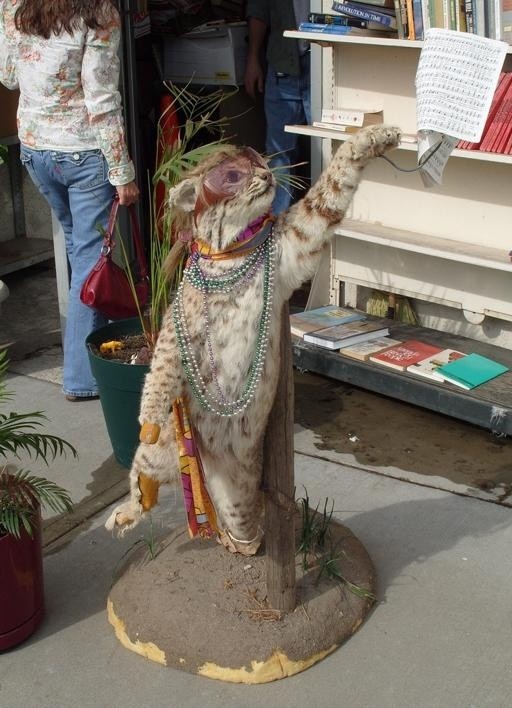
top-left (0, 334), bottom-right (79, 657)
top-left (86, 69), bottom-right (305, 472)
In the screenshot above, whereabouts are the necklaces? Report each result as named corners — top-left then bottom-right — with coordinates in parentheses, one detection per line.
top-left (171, 226), bottom-right (276, 420)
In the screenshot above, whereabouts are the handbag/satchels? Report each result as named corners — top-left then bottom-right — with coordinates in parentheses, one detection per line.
top-left (79, 252), bottom-right (150, 318)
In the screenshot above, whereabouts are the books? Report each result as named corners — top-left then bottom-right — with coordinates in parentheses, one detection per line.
top-left (288, 303), bottom-right (510, 391)
top-left (299, 0), bottom-right (511, 46)
top-left (313, 109), bottom-right (417, 143)
top-left (455, 72), bottom-right (512, 155)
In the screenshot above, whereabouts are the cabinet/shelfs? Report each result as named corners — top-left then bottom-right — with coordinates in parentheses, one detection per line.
top-left (282, 29), bottom-right (512, 444)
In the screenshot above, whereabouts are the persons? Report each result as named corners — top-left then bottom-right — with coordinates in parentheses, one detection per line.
top-left (241, 0), bottom-right (312, 212)
top-left (2, 0), bottom-right (142, 404)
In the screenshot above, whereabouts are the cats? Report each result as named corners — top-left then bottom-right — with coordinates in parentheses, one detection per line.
top-left (102, 125), bottom-right (405, 553)
